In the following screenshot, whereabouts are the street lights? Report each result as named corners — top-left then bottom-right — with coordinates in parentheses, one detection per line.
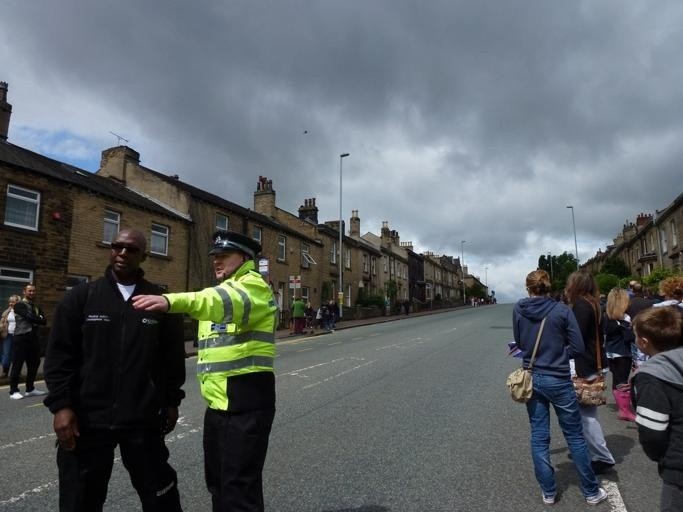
top-left (460, 239), bottom-right (467, 304)
top-left (337, 150), bottom-right (350, 318)
top-left (484, 267), bottom-right (490, 286)
top-left (565, 205), bottom-right (578, 262)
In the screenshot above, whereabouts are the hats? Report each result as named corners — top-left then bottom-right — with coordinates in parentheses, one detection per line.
top-left (208, 231), bottom-right (262, 258)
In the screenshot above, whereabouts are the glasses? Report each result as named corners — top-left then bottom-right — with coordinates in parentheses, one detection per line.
top-left (111, 243), bottom-right (139, 252)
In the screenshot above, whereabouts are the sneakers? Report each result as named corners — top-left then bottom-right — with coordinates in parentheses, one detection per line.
top-left (25, 389), bottom-right (45, 396)
top-left (542, 490), bottom-right (555, 505)
top-left (586, 488), bottom-right (607, 505)
top-left (10, 392), bottom-right (23, 400)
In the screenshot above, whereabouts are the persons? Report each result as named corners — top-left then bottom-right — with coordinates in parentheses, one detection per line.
top-left (45, 229), bottom-right (184, 512)
top-left (289, 296), bottom-right (339, 337)
top-left (630, 306), bottom-right (682, 512)
top-left (511, 279), bottom-right (683, 428)
top-left (130, 231), bottom-right (277, 512)
top-left (0, 295), bottom-right (21, 378)
top-left (401, 298), bottom-right (412, 317)
top-left (8, 283), bottom-right (45, 400)
top-left (463, 293), bottom-right (499, 309)
top-left (561, 269), bottom-right (615, 474)
top-left (511, 270), bottom-right (607, 504)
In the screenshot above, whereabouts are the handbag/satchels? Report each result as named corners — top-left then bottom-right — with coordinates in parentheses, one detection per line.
top-left (572, 370), bottom-right (606, 406)
top-left (507, 368), bottom-right (533, 402)
